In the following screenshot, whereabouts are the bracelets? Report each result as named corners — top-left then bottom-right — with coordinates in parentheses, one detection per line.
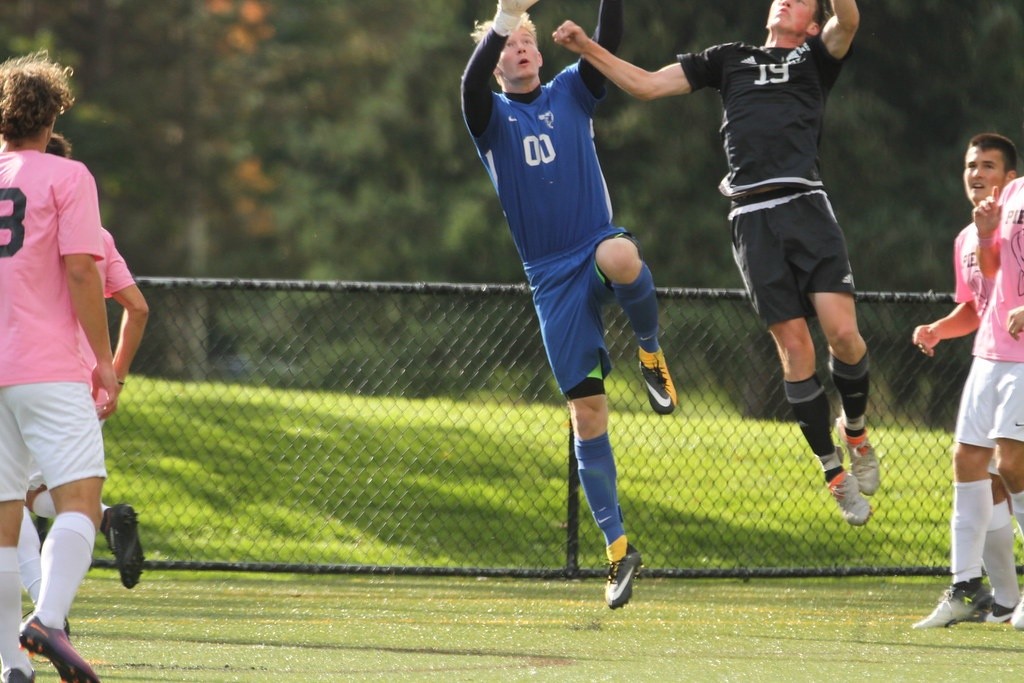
top-left (119, 381), bottom-right (124, 385)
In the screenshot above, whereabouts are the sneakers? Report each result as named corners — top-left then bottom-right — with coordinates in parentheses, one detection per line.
top-left (833, 416), bottom-right (879, 495)
top-left (913, 576), bottom-right (994, 629)
top-left (3, 617), bottom-right (101, 683)
top-left (605, 543), bottom-right (643, 610)
top-left (100, 504), bottom-right (145, 589)
top-left (966, 604), bottom-right (1020, 623)
top-left (1011, 595), bottom-right (1024, 628)
top-left (831, 471), bottom-right (872, 526)
top-left (639, 360), bottom-right (677, 414)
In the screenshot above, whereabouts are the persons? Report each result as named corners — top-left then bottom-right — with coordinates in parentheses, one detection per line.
top-left (911, 133), bottom-right (1017, 628)
top-left (552, 0), bottom-right (881, 531)
top-left (914, 172), bottom-right (1024, 634)
top-left (461, 1), bottom-right (681, 613)
top-left (39, 136), bottom-right (152, 586)
top-left (0, 49), bottom-right (120, 683)
